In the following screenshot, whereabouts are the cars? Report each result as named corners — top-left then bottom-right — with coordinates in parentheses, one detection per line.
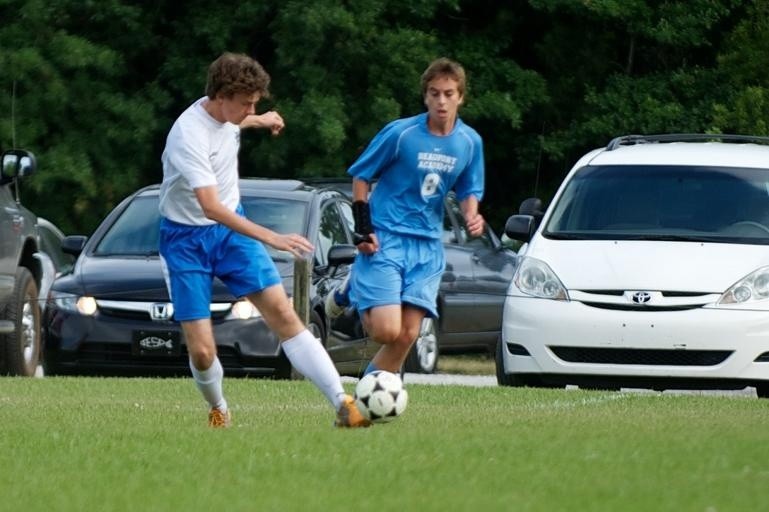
top-left (496, 133), bottom-right (769, 398)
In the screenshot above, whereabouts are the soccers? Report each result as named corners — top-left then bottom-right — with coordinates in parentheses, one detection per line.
top-left (354, 370), bottom-right (406, 422)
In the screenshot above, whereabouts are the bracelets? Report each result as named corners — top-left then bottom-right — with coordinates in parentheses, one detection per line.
top-left (351, 201), bottom-right (374, 235)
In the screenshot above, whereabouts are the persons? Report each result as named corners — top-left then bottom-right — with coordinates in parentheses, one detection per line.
top-left (349, 58), bottom-right (487, 404)
top-left (157, 50), bottom-right (370, 426)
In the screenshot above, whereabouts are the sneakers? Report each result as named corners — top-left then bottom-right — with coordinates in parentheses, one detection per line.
top-left (333, 395), bottom-right (374, 429)
top-left (324, 271), bottom-right (351, 320)
top-left (208, 407), bottom-right (231, 431)
